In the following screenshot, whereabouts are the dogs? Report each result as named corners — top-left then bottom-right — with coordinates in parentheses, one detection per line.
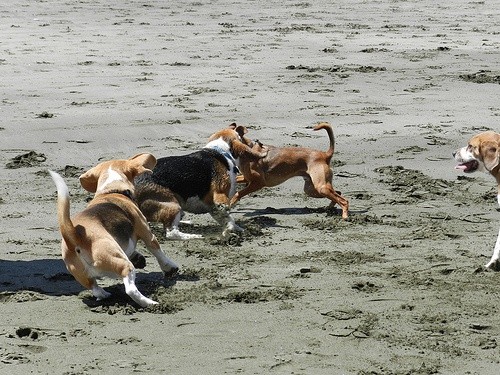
top-left (451, 130), bottom-right (500, 274)
top-left (229, 122), bottom-right (350, 222)
top-left (131, 127), bottom-right (269, 239)
top-left (46, 152), bottom-right (184, 310)
